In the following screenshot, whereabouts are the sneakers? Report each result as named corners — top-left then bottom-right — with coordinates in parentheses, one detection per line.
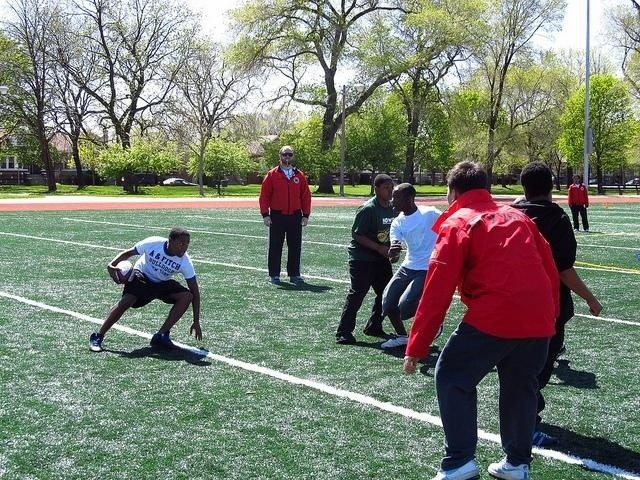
top-left (381, 333), bottom-right (409, 350)
top-left (531, 431), bottom-right (559, 446)
top-left (267, 276), bottom-right (280, 285)
top-left (432, 324), bottom-right (443, 341)
top-left (486, 457), bottom-right (529, 480)
top-left (88, 333), bottom-right (103, 353)
top-left (290, 275), bottom-right (305, 285)
top-left (431, 458), bottom-right (480, 480)
top-left (150, 331), bottom-right (182, 352)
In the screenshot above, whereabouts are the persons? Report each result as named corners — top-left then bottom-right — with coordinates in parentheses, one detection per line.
top-left (88, 227), bottom-right (203, 352)
top-left (259, 146), bottom-right (311, 284)
top-left (568, 174), bottom-right (590, 232)
top-left (380, 182), bottom-right (443, 348)
top-left (402, 161), bottom-right (560, 480)
top-left (509, 162), bottom-right (603, 447)
top-left (335, 173), bottom-right (397, 344)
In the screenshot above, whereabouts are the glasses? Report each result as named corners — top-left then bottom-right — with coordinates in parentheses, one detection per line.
top-left (279, 151), bottom-right (294, 159)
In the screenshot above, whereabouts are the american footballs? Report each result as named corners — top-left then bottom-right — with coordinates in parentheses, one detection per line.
top-left (114, 260), bottom-right (133, 282)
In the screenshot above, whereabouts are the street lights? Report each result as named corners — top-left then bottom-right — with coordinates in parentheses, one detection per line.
top-left (340, 83), bottom-right (364, 196)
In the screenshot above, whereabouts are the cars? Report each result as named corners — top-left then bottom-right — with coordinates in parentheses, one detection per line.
top-left (163, 178), bottom-right (198, 186)
